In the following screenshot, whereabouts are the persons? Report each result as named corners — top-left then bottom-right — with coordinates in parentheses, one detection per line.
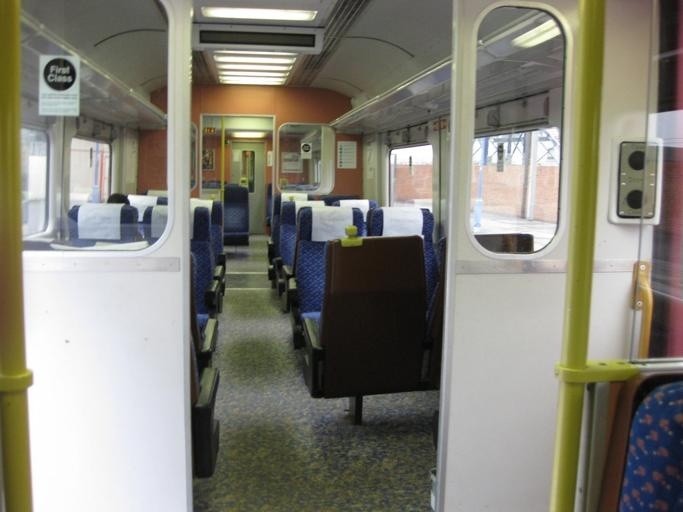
top-left (106, 192), bottom-right (143, 240)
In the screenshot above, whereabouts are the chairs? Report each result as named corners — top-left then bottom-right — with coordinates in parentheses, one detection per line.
top-left (266, 182), bottom-right (538, 425)
top-left (66, 179), bottom-right (249, 479)
